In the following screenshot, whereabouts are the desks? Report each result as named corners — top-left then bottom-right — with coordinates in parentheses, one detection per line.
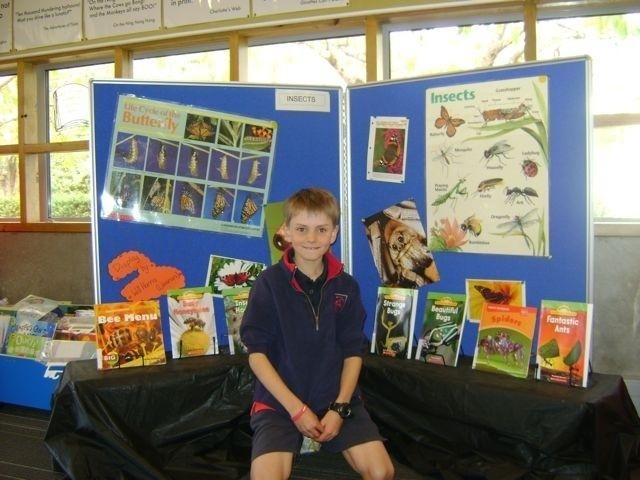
top-left (47, 358), bottom-right (638, 478)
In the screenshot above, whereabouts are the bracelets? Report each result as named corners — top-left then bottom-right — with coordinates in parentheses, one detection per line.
top-left (290, 405), bottom-right (308, 423)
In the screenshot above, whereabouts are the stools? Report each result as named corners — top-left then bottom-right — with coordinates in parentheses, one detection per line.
top-left (226, 405), bottom-right (393, 479)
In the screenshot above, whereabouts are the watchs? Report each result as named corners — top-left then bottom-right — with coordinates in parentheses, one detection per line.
top-left (328, 402), bottom-right (352, 420)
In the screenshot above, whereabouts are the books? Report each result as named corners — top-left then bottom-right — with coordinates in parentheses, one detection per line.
top-left (222, 287), bottom-right (251, 355)
top-left (166, 286), bottom-right (219, 359)
top-left (94, 300), bottom-right (167, 371)
top-left (415, 292), bottom-right (467, 368)
top-left (370, 287), bottom-right (419, 360)
top-left (472, 303), bottom-right (537, 379)
top-left (534, 298), bottom-right (594, 389)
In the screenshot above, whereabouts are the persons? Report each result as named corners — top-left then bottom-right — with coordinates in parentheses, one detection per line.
top-left (240, 191), bottom-right (395, 480)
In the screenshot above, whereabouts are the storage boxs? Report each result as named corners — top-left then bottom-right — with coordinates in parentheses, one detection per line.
top-left (1, 354), bottom-right (97, 413)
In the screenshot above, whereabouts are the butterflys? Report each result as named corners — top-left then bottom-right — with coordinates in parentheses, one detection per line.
top-left (219, 271), bottom-right (252, 286)
top-left (434, 106), bottom-right (465, 138)
